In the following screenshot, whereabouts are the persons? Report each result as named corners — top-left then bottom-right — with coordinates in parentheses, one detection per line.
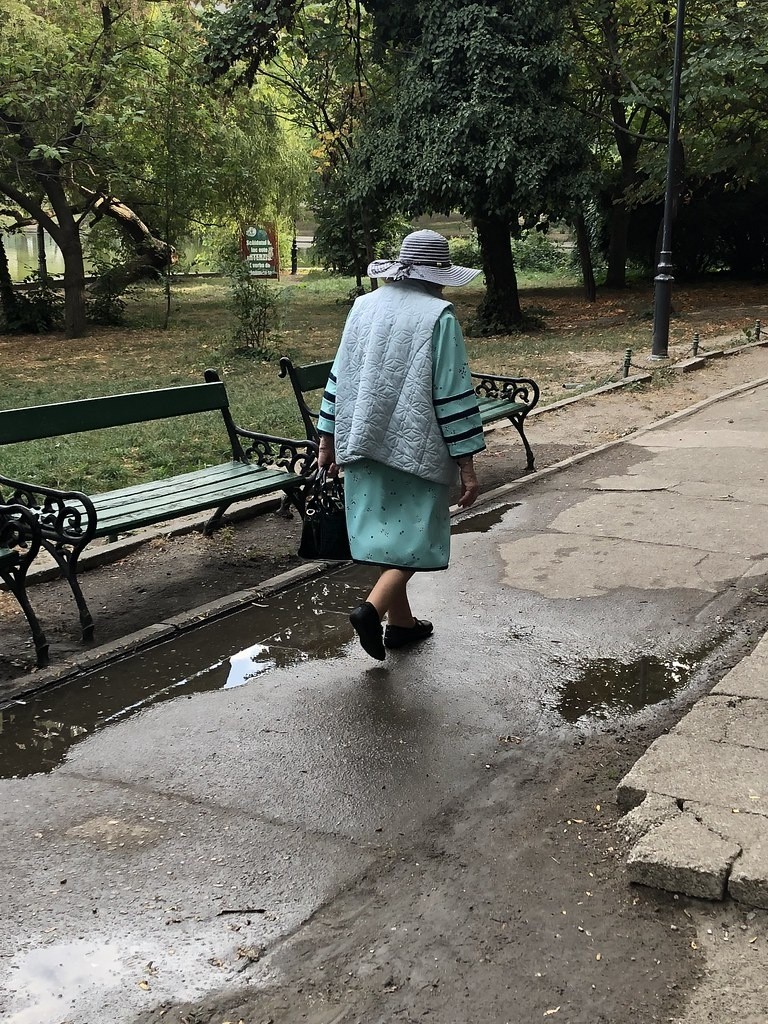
top-left (315, 229), bottom-right (487, 662)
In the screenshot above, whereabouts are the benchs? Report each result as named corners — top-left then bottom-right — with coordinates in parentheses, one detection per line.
top-left (0, 357), bottom-right (540, 669)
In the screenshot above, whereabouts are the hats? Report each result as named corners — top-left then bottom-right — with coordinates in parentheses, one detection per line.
top-left (367, 229), bottom-right (483, 287)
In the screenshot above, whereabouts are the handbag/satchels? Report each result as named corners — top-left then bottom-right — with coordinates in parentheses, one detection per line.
top-left (297, 466), bottom-right (352, 560)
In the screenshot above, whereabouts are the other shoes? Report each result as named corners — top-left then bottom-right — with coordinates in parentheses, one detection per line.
top-left (349, 602), bottom-right (386, 661)
top-left (384, 617), bottom-right (433, 648)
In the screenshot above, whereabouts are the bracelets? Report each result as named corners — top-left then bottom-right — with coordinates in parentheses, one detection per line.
top-left (460, 457), bottom-right (473, 468)
